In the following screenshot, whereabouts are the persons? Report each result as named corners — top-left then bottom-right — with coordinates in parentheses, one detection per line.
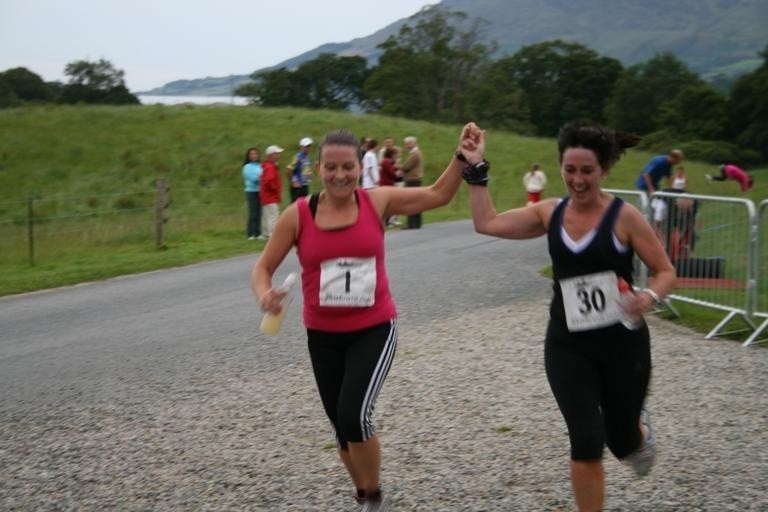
top-left (522, 164), bottom-right (547, 205)
top-left (242, 134), bottom-right (422, 241)
top-left (704, 164), bottom-right (754, 193)
top-left (635, 148), bottom-right (698, 258)
top-left (457, 118), bottom-right (677, 512)
top-left (252, 121), bottom-right (486, 512)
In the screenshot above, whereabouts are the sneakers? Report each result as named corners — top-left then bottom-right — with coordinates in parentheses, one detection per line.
top-left (246, 233), bottom-right (272, 240)
top-left (387, 218), bottom-right (402, 229)
top-left (356, 489), bottom-right (386, 512)
top-left (633, 409), bottom-right (656, 475)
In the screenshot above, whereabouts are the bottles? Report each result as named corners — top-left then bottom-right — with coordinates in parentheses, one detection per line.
top-left (259, 272), bottom-right (299, 337)
top-left (616, 275), bottom-right (643, 331)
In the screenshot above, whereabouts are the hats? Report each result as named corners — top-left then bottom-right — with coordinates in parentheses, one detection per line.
top-left (264, 144), bottom-right (285, 156)
top-left (299, 138), bottom-right (314, 148)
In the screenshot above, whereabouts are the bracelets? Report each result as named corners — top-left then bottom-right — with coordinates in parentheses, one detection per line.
top-left (642, 287), bottom-right (663, 306)
top-left (454, 150), bottom-right (469, 164)
